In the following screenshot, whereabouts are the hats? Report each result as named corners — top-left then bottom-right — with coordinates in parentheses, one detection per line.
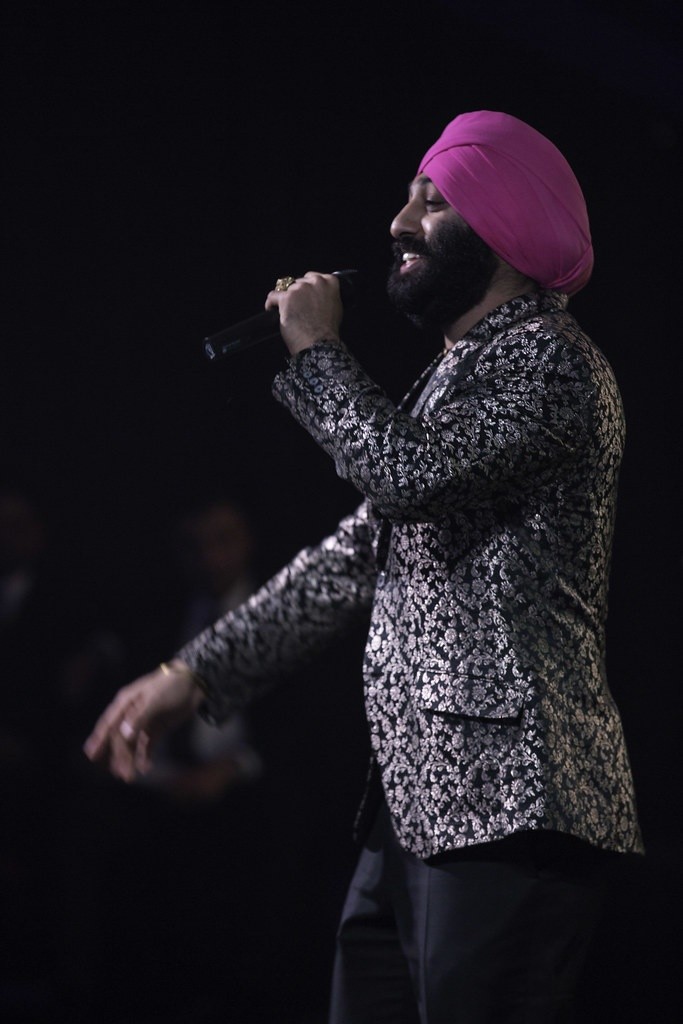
top-left (416, 109), bottom-right (593, 294)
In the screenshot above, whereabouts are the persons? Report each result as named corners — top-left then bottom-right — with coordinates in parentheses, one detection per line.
top-left (84, 110), bottom-right (644, 1024)
top-left (0, 474), bottom-right (326, 1024)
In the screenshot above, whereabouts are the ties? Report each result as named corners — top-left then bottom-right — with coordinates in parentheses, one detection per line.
top-left (200, 591), bottom-right (221, 632)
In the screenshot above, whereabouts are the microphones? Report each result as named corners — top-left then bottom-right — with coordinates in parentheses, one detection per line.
top-left (202, 270), bottom-right (366, 362)
